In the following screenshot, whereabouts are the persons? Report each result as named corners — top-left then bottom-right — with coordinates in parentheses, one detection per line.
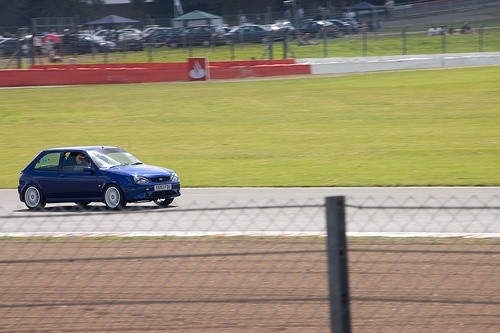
top-left (75, 153), bottom-right (87, 166)
top-left (426, 24), bottom-right (453, 35)
top-left (461, 22), bottom-right (470, 34)
top-left (20, 42), bottom-right (32, 57)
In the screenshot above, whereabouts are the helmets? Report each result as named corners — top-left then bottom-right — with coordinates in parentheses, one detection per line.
top-left (75, 154), bottom-right (87, 165)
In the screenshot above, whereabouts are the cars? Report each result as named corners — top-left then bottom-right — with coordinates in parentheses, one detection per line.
top-left (17, 146), bottom-right (180, 210)
top-left (0, 8), bottom-right (385, 62)
